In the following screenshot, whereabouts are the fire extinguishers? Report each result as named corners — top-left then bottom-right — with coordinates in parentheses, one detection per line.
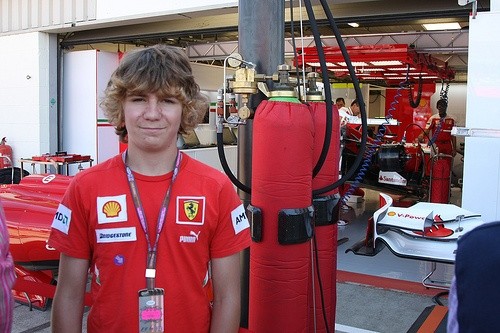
top-left (0, 137), bottom-right (13, 170)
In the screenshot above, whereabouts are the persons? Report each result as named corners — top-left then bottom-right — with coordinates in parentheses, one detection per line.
top-left (447, 220), bottom-right (500, 333)
top-left (47, 45), bottom-right (254, 332)
top-left (336, 98), bottom-right (457, 171)
